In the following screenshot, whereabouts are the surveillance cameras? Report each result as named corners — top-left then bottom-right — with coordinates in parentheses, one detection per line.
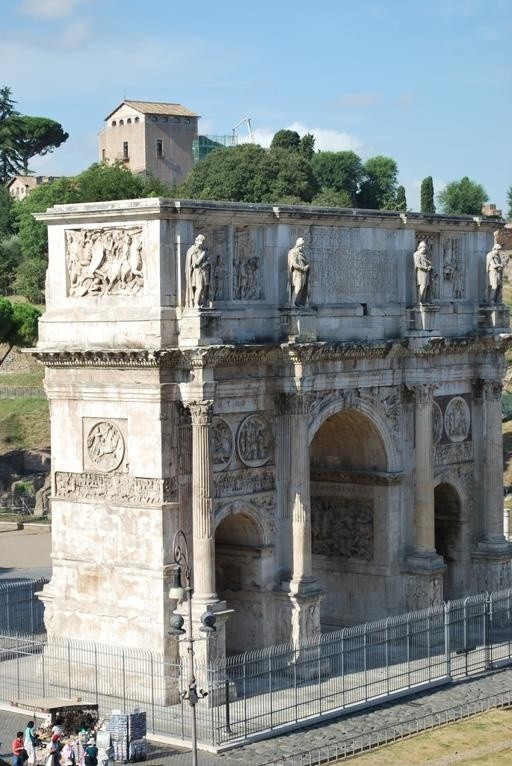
top-left (200, 688), bottom-right (208, 697)
top-left (178, 690), bottom-right (187, 696)
top-left (189, 683), bottom-right (196, 693)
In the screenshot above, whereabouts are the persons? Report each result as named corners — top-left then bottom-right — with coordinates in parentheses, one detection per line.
top-left (287, 238), bottom-right (311, 309)
top-left (406, 575), bottom-right (441, 612)
top-left (185, 233), bottom-right (213, 310)
top-left (11, 712), bottom-right (98, 766)
top-left (413, 240), bottom-right (432, 304)
top-left (485, 243), bottom-right (507, 305)
top-left (306, 606), bottom-right (315, 637)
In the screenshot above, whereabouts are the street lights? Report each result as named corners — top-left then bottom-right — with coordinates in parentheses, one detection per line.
top-left (169, 526), bottom-right (217, 765)
